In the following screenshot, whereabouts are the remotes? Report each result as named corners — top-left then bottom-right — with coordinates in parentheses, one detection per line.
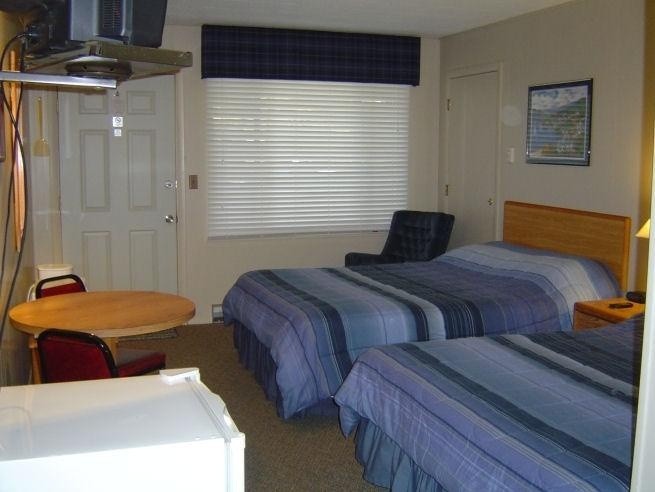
top-left (608, 301), bottom-right (634, 309)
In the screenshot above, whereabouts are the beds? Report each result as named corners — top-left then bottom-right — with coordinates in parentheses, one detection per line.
top-left (222, 199), bottom-right (631, 424)
top-left (334, 313), bottom-right (643, 492)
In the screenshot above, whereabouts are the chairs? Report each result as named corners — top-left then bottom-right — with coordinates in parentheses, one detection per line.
top-left (37, 328), bottom-right (165, 382)
top-left (36, 274), bottom-right (86, 299)
top-left (346, 210), bottom-right (454, 266)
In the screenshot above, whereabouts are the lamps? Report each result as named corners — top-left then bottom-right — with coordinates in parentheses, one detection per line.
top-left (627, 216), bottom-right (651, 303)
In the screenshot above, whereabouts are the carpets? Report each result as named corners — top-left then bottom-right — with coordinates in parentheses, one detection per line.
top-left (117, 328), bottom-right (179, 342)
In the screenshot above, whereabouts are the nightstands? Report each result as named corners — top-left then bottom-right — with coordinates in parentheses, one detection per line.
top-left (572, 297), bottom-right (645, 334)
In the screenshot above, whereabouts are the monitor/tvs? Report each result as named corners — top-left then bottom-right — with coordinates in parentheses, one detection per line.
top-left (17, 1), bottom-right (168, 61)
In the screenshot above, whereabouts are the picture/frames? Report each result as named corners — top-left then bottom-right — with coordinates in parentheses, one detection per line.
top-left (525, 79), bottom-right (593, 166)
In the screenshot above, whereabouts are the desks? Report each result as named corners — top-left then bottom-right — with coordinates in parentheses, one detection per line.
top-left (8, 291), bottom-right (196, 384)
